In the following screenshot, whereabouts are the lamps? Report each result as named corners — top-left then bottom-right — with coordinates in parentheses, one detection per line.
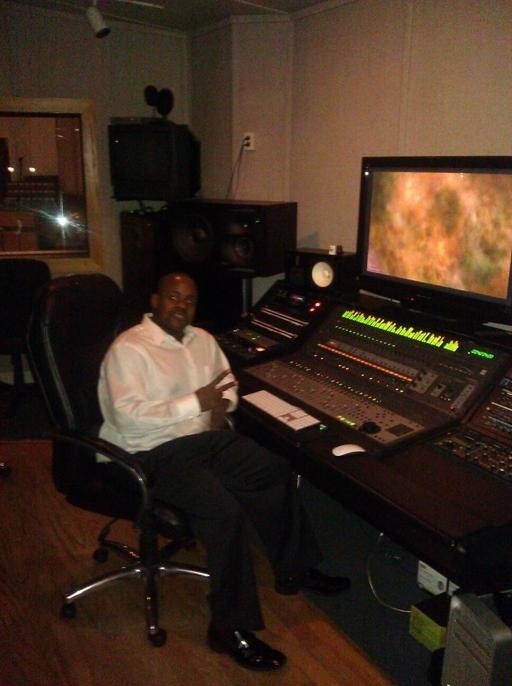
top-left (85, 0), bottom-right (110, 38)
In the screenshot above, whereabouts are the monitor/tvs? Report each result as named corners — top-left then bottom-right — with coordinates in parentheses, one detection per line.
top-left (355, 154), bottom-right (511, 326)
top-left (107, 121), bottom-right (201, 201)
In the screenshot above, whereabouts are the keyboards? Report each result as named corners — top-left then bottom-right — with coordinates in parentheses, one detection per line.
top-left (240, 389), bottom-right (322, 432)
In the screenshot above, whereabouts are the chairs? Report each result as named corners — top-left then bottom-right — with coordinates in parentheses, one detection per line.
top-left (25, 272), bottom-right (245, 647)
top-left (0, 258), bottom-right (51, 406)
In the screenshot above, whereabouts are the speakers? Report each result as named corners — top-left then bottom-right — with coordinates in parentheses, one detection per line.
top-left (161, 196), bottom-right (298, 277)
top-left (282, 246), bottom-right (356, 296)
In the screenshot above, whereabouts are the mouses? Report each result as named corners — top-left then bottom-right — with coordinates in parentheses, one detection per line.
top-left (331, 443), bottom-right (367, 458)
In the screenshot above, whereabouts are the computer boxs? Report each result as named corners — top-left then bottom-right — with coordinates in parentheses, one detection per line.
top-left (441, 586), bottom-right (512, 686)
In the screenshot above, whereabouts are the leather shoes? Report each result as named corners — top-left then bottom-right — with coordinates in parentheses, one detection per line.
top-left (207, 629), bottom-right (286, 670)
top-left (275, 570), bottom-right (351, 595)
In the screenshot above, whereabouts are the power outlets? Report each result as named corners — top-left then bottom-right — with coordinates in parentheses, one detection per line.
top-left (243, 133), bottom-right (254, 151)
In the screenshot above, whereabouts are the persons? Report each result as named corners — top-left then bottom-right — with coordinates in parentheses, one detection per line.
top-left (91, 269), bottom-right (354, 673)
